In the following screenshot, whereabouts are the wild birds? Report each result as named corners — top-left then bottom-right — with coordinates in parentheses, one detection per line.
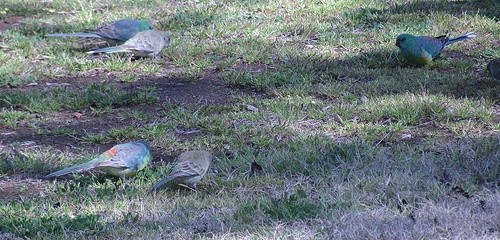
top-left (395, 30), bottom-right (477, 66)
top-left (38, 139), bottom-right (151, 184)
top-left (87, 29), bottom-right (171, 60)
top-left (43, 18), bottom-right (154, 45)
top-left (149, 149), bottom-right (215, 191)
